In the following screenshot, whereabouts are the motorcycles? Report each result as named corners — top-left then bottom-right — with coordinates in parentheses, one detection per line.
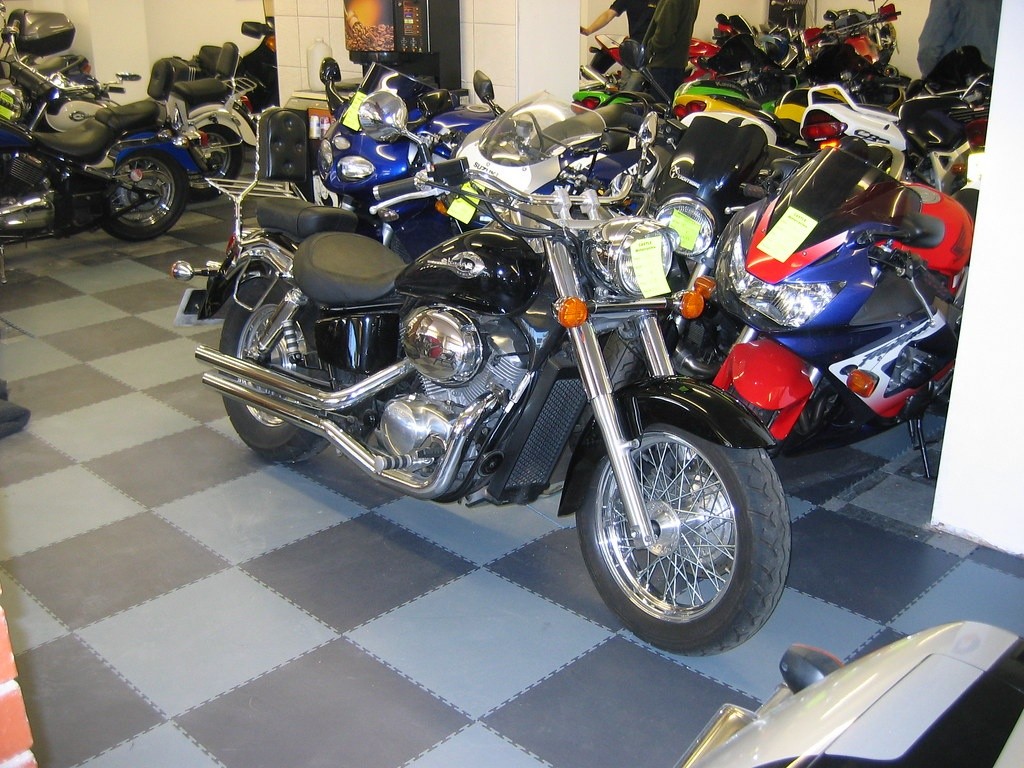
top-left (170, 107), bottom-right (792, 658)
top-left (1, 1), bottom-right (92, 123)
top-left (0, 20), bottom-right (208, 283)
top-left (312, 0), bottom-right (991, 482)
top-left (0, 16), bottom-right (256, 203)
top-left (191, 16), bottom-right (280, 113)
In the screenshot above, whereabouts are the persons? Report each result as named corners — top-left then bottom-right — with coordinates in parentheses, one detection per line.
top-left (580, 0), bottom-right (656, 58)
top-left (640, 0), bottom-right (699, 106)
top-left (919, 0), bottom-right (1001, 83)
